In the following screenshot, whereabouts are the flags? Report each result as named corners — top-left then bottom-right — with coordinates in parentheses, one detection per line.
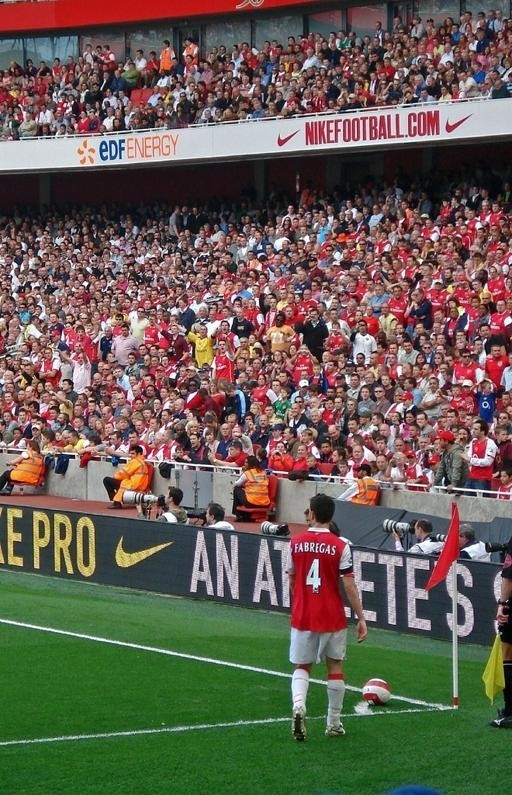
top-left (481, 630), bottom-right (507, 708)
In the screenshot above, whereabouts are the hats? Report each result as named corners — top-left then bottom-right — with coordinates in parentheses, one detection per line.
top-left (31, 425), bottom-right (40, 429)
top-left (186, 365), bottom-right (195, 372)
top-left (299, 380), bottom-right (309, 387)
top-left (406, 451), bottom-right (416, 457)
top-left (435, 432), bottom-right (455, 443)
top-left (462, 379), bottom-right (473, 387)
top-left (357, 464), bottom-right (371, 472)
top-left (428, 456), bottom-right (440, 465)
top-left (399, 393), bottom-right (413, 401)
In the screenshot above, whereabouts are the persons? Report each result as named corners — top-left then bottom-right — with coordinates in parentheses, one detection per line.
top-left (192, 502), bottom-right (234, 530)
top-left (136, 486), bottom-right (187, 523)
top-left (103, 445), bottom-right (149, 509)
top-left (304, 509), bottom-right (312, 528)
top-left (487, 534), bottom-right (512, 730)
top-left (2, 440), bottom-right (44, 495)
top-left (281, 494), bottom-right (370, 743)
top-left (444, 523), bottom-right (491, 560)
top-left (392, 519), bottom-right (445, 554)
top-left (232, 456), bottom-right (270, 522)
top-left (1, 8), bottom-right (511, 142)
top-left (336, 464), bottom-right (379, 506)
top-left (329, 521), bottom-right (353, 544)
top-left (3, 168), bottom-right (511, 498)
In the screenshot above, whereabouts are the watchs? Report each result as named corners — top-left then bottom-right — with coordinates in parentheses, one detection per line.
top-left (496, 599), bottom-right (509, 604)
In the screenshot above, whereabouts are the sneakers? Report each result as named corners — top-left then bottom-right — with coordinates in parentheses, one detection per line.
top-left (108, 503), bottom-right (121, 509)
top-left (489, 716), bottom-right (512, 728)
top-left (292, 704), bottom-right (307, 741)
top-left (325, 724), bottom-right (345, 736)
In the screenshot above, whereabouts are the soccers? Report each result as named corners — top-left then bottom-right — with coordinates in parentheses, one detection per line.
top-left (361, 676), bottom-right (391, 706)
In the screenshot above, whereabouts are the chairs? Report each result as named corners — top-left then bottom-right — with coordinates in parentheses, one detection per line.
top-left (235, 475), bottom-right (280, 523)
top-left (145, 462), bottom-right (154, 492)
top-left (129, 88), bottom-right (155, 107)
top-left (11, 454), bottom-right (50, 494)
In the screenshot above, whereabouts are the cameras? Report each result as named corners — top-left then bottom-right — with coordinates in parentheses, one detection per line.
top-left (485, 542), bottom-right (509, 555)
top-left (187, 510), bottom-right (206, 526)
